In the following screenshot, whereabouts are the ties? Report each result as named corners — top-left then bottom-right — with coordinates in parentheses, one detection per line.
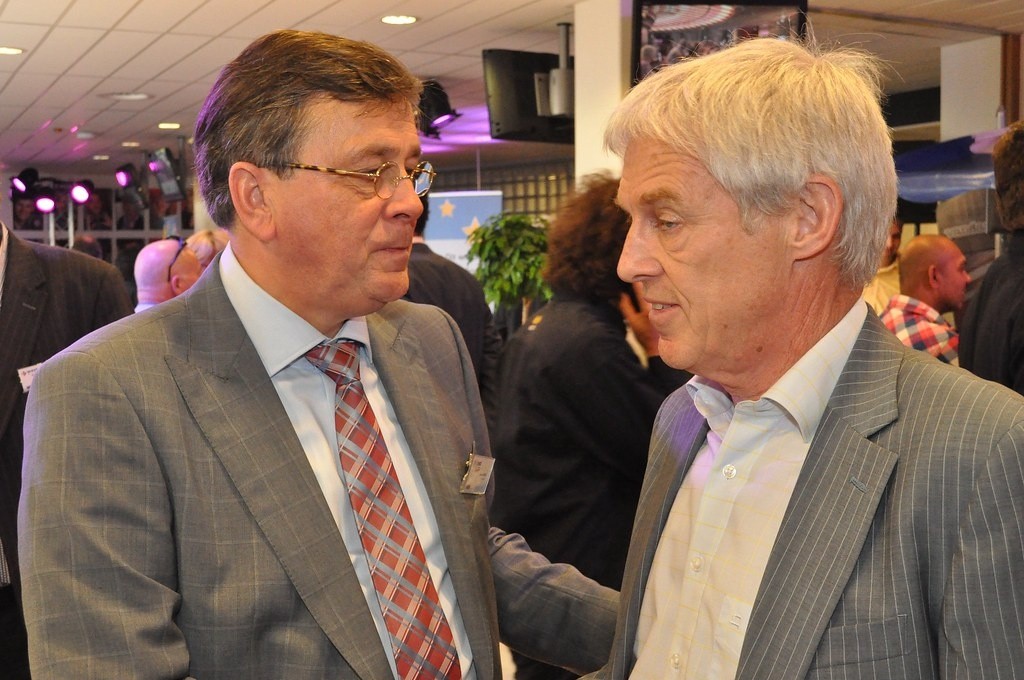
top-left (303, 339), bottom-right (463, 680)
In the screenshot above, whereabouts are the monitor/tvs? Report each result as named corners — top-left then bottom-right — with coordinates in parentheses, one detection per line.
top-left (150, 147), bottom-right (187, 203)
top-left (629, 0), bottom-right (807, 90)
top-left (482, 49), bottom-right (575, 144)
top-left (117, 164), bottom-right (151, 210)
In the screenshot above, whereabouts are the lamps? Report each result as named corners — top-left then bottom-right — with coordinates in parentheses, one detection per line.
top-left (12, 147), bottom-right (186, 227)
top-left (418, 81), bottom-right (460, 130)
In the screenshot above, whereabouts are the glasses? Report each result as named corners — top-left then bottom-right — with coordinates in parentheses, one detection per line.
top-left (265, 161), bottom-right (436, 199)
top-left (165, 235), bottom-right (187, 282)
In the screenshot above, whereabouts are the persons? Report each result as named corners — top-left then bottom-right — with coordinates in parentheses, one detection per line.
top-left (402, 192), bottom-right (503, 438)
top-left (641, 27), bottom-right (757, 78)
top-left (71, 235), bottom-right (103, 260)
top-left (491, 171), bottom-right (696, 680)
top-left (134, 231), bottom-right (230, 316)
top-left (959, 120), bottom-right (1024, 397)
top-left (0, 221), bottom-right (136, 680)
top-left (574, 36), bottom-right (1024, 680)
top-left (17, 29), bottom-right (621, 680)
top-left (876, 232), bottom-right (972, 369)
top-left (860, 215), bottom-right (904, 319)
